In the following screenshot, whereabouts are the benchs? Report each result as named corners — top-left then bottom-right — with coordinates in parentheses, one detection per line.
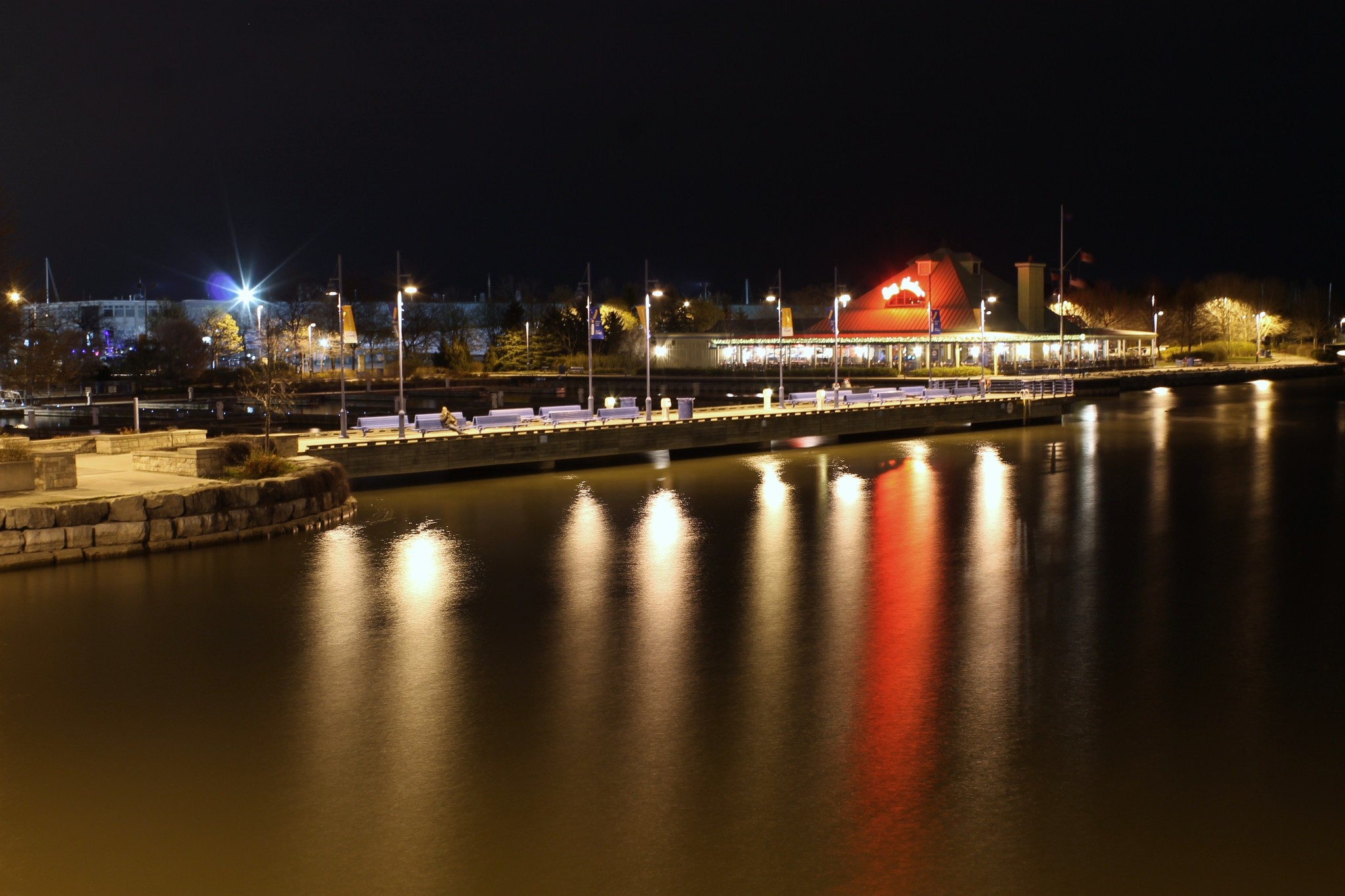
top-left (784, 386), bottom-right (984, 412)
top-left (349, 405), bottom-right (647, 438)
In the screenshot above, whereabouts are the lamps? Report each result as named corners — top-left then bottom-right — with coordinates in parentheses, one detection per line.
top-left (604, 396), bottom-right (615, 410)
top-left (816, 388), bottom-right (826, 411)
top-left (762, 388), bottom-right (773, 410)
top-left (660, 397), bottom-right (672, 422)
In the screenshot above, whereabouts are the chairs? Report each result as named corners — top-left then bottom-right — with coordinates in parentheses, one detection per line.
top-left (723, 352), bottom-right (1151, 374)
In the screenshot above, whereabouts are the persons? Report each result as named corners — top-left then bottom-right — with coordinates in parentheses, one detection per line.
top-left (1255, 351), bottom-right (1259, 362)
top-left (441, 406), bottom-right (468, 436)
top-left (1182, 357), bottom-right (1188, 366)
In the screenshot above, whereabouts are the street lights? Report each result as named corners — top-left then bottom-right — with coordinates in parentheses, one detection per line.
top-left (645, 278), bottom-right (663, 421)
top-left (834, 284), bottom-right (851, 407)
top-left (981, 288), bottom-right (996, 398)
top-left (326, 278), bottom-right (347, 437)
top-left (257, 305), bottom-right (264, 358)
top-left (574, 282), bottom-right (594, 417)
top-left (308, 323), bottom-right (315, 373)
top-left (916, 289), bottom-right (932, 393)
top-left (397, 274), bottom-right (417, 436)
top-left (1340, 318), bottom-right (1345, 342)
top-left (766, 286), bottom-right (784, 409)
top-left (1053, 288), bottom-right (1065, 377)
top-left (1153, 306), bottom-right (1164, 365)
